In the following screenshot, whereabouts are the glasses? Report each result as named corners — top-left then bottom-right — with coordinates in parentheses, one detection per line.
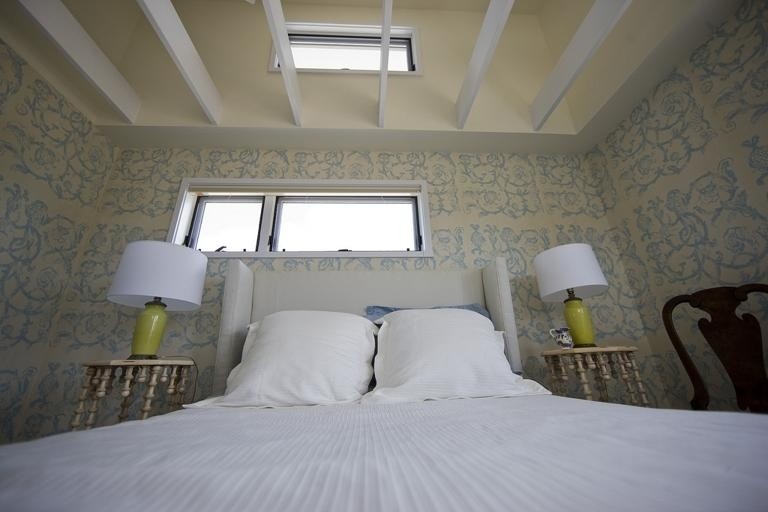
top-left (533, 241), bottom-right (611, 350)
top-left (104, 238), bottom-right (210, 361)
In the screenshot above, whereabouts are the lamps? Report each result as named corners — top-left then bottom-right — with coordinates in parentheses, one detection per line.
top-left (659, 281), bottom-right (767, 416)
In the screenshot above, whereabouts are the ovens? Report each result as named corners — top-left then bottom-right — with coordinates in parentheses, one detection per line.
top-left (1, 259), bottom-right (766, 511)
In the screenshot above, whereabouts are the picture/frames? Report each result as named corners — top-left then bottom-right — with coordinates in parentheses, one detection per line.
top-left (536, 344), bottom-right (650, 408)
top-left (68, 357), bottom-right (195, 435)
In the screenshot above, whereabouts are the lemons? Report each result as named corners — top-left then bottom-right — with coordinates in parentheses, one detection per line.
top-left (548, 326), bottom-right (575, 351)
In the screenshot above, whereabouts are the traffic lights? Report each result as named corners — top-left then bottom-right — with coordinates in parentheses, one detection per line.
top-left (358, 307), bottom-right (553, 410)
top-left (365, 302), bottom-right (494, 323)
top-left (180, 308), bottom-right (381, 410)
